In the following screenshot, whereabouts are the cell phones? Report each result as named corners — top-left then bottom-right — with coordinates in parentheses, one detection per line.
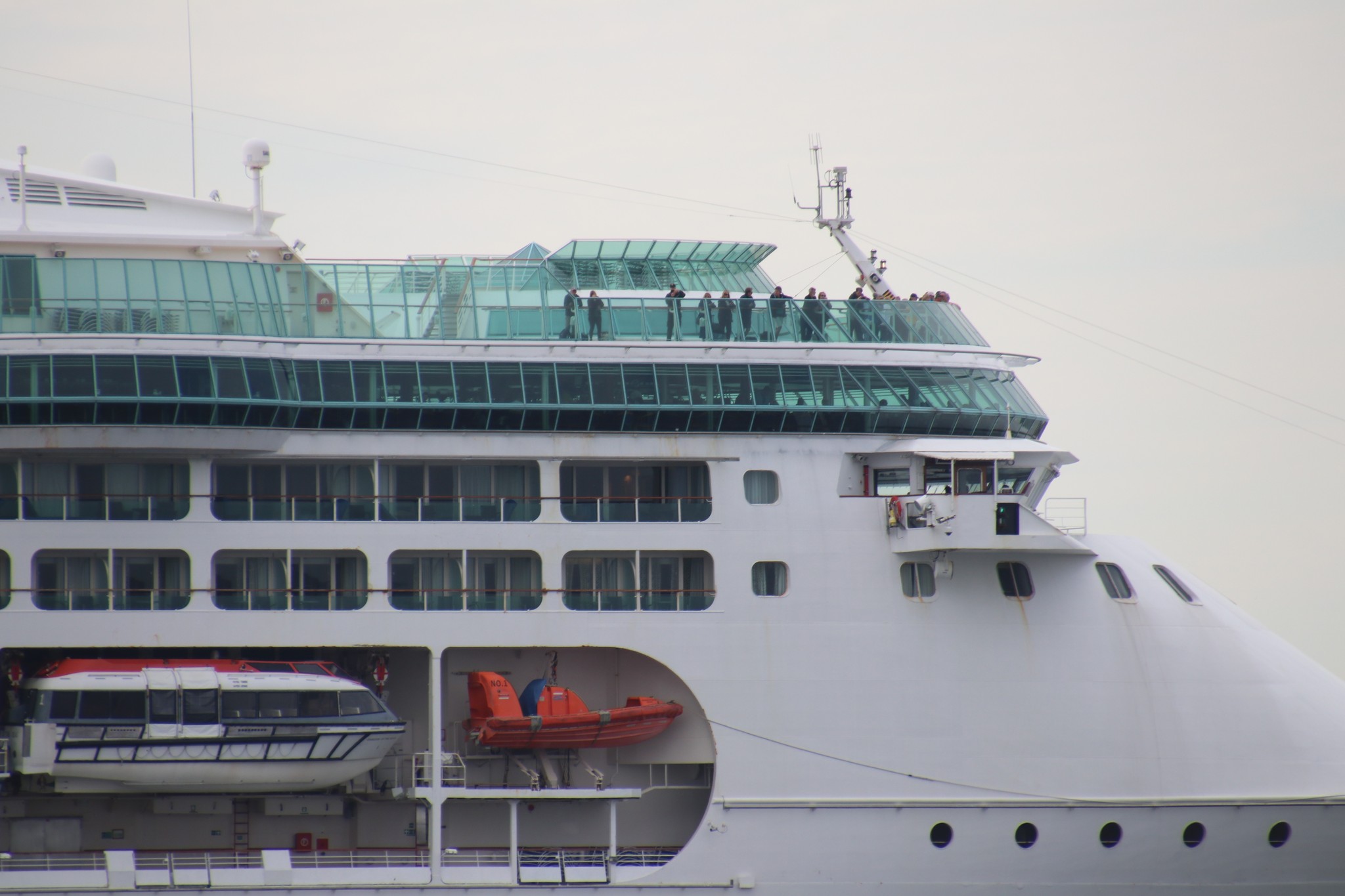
top-left (671, 290), bottom-right (674, 292)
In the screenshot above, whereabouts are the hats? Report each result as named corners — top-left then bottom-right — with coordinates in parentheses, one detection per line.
top-left (670, 284), bottom-right (676, 288)
top-left (938, 292), bottom-right (945, 296)
top-left (927, 291), bottom-right (935, 296)
top-left (911, 293), bottom-right (919, 299)
top-left (571, 287), bottom-right (576, 291)
top-left (873, 292), bottom-right (880, 298)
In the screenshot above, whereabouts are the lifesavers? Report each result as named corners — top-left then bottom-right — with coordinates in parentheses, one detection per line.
top-left (890, 495), bottom-right (902, 521)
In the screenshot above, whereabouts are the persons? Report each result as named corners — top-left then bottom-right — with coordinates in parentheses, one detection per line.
top-left (389, 365), bottom-right (1028, 439)
top-left (861, 292), bottom-right (962, 343)
top-left (738, 287), bottom-right (756, 341)
top-left (1003, 484), bottom-right (1011, 493)
top-left (944, 485), bottom-right (952, 494)
top-left (799, 287), bottom-right (819, 343)
top-left (814, 292), bottom-right (833, 343)
top-left (770, 286), bottom-right (793, 342)
top-left (664, 284), bottom-right (686, 341)
top-left (846, 286), bottom-right (871, 343)
top-left (587, 291), bottom-right (605, 341)
top-left (696, 293), bottom-right (717, 341)
top-left (717, 290), bottom-right (737, 342)
top-left (564, 288), bottom-right (584, 340)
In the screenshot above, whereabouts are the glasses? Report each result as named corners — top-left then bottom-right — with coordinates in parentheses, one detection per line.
top-left (724, 294), bottom-right (729, 296)
top-left (820, 295), bottom-right (826, 297)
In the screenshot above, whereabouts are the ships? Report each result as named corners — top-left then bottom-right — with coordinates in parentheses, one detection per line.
top-left (0, 132), bottom-right (1345, 896)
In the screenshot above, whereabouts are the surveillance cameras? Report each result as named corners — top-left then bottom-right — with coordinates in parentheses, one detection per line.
top-left (944, 521), bottom-right (952, 536)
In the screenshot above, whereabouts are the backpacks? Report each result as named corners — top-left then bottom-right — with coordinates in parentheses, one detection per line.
top-left (581, 333), bottom-right (589, 340)
top-left (559, 328), bottom-right (571, 339)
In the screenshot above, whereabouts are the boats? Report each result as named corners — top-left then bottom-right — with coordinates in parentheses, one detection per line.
top-left (462, 672), bottom-right (687, 757)
top-left (8, 657), bottom-right (408, 794)
